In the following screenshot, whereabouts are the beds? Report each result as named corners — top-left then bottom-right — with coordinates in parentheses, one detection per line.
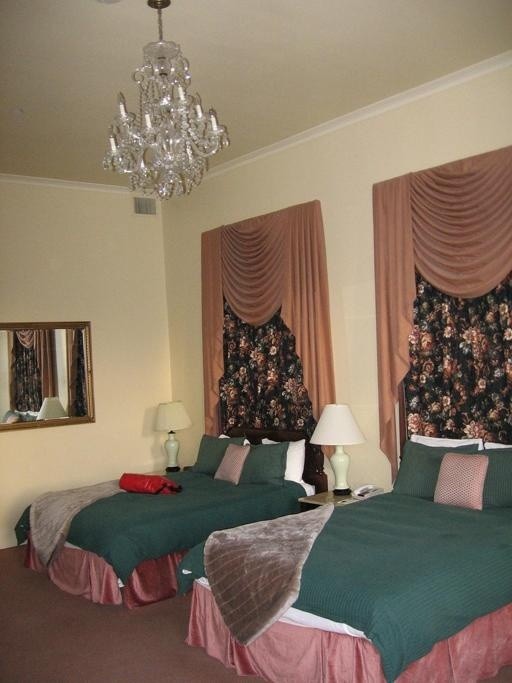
top-left (175, 491), bottom-right (512, 683)
top-left (14, 427), bottom-right (327, 612)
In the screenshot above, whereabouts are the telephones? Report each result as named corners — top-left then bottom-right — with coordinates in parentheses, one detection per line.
top-left (351, 485), bottom-right (384, 500)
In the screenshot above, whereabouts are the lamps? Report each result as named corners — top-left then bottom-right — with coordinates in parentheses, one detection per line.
top-left (308, 404), bottom-right (366, 495)
top-left (36, 396), bottom-right (70, 421)
top-left (153, 401), bottom-right (192, 472)
top-left (102, 1), bottom-right (230, 202)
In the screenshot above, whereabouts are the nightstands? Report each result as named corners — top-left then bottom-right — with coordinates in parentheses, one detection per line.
top-left (297, 491), bottom-right (352, 513)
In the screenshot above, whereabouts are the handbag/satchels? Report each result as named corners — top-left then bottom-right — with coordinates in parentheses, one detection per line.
top-left (118, 472), bottom-right (186, 495)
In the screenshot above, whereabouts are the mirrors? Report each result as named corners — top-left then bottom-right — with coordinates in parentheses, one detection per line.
top-left (0, 321), bottom-right (95, 432)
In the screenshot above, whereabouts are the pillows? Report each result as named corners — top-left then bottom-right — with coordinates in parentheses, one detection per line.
top-left (391, 440), bottom-right (479, 500)
top-left (433, 452), bottom-right (489, 511)
top-left (213, 443), bottom-right (251, 486)
top-left (410, 435), bottom-right (485, 450)
top-left (3, 410), bottom-right (36, 423)
top-left (483, 442), bottom-right (512, 449)
top-left (191, 435), bottom-right (246, 474)
top-left (477, 448), bottom-right (512, 509)
top-left (262, 438), bottom-right (306, 482)
top-left (218, 433), bottom-right (251, 446)
top-left (239, 441), bottom-right (289, 487)
